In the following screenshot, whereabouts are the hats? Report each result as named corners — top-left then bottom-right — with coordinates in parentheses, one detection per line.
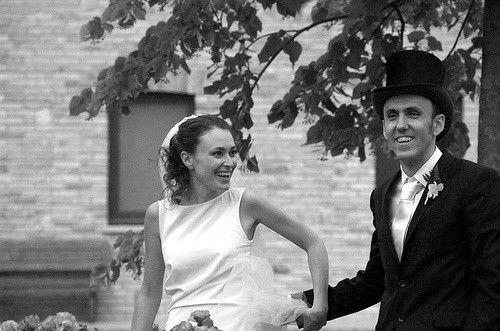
top-left (371, 50), bottom-right (454, 142)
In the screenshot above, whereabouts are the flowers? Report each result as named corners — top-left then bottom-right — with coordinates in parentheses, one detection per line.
top-left (422, 164), bottom-right (444, 205)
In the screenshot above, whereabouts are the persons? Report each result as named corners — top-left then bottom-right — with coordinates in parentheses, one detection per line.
top-left (130, 114), bottom-right (328, 331)
top-left (286, 50), bottom-right (500, 330)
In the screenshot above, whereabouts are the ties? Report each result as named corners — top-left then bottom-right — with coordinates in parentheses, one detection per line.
top-left (391, 181), bottom-right (423, 263)
